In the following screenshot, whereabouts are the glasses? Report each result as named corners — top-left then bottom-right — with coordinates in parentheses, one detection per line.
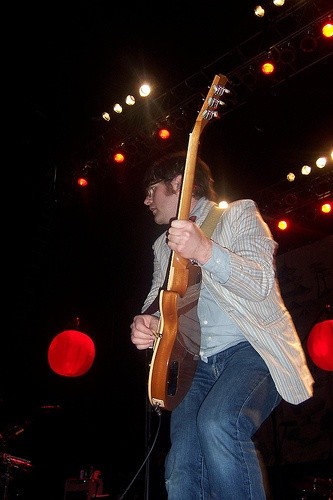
top-left (146, 179), bottom-right (161, 198)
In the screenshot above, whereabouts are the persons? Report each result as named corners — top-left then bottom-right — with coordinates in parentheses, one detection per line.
top-left (128, 151), bottom-right (316, 499)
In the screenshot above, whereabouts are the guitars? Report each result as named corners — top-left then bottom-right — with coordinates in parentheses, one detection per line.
top-left (146, 72), bottom-right (230, 411)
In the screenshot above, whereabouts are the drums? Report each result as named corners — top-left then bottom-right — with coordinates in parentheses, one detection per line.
top-left (295, 475), bottom-right (332, 497)
top-left (0, 452), bottom-right (32, 474)
